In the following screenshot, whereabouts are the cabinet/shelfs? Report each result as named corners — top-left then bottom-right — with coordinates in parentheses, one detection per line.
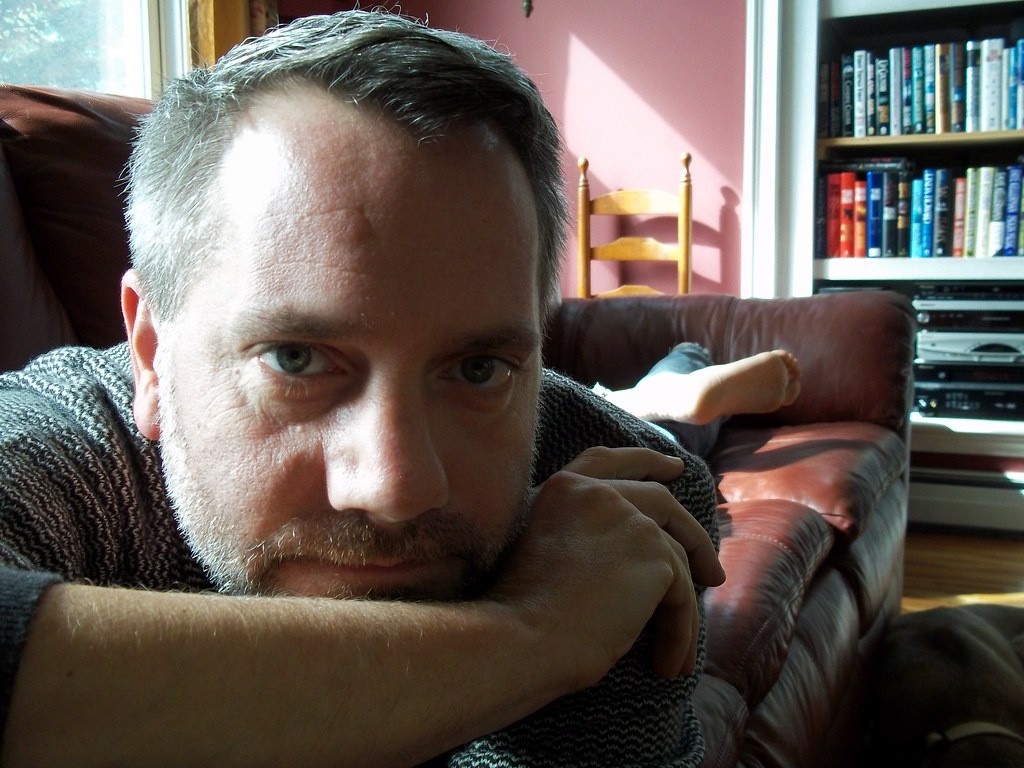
top-left (810, 0), bottom-right (1024, 280)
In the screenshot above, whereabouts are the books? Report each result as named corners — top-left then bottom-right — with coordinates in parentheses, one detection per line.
top-left (816, 38), bottom-right (1024, 137)
top-left (815, 159), bottom-right (1024, 260)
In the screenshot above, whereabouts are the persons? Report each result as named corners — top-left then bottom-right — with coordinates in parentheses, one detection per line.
top-left (0, 10), bottom-right (804, 768)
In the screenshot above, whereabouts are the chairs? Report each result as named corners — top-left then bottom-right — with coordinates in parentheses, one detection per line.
top-left (576, 153), bottom-right (694, 297)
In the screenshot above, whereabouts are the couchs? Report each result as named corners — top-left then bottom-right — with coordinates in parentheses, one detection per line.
top-left (0, 81), bottom-right (918, 768)
